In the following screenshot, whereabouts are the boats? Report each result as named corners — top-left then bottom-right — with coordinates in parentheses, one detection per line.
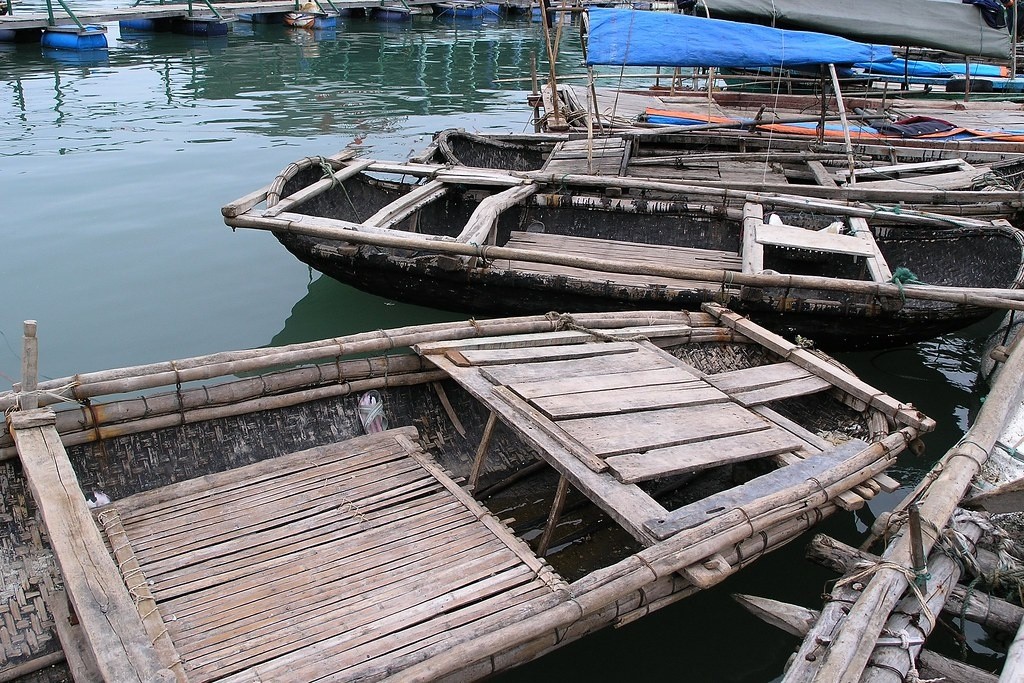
top-left (236, 14), bottom-right (337, 29)
top-left (437, 1), bottom-right (558, 22)
top-left (492, 0), bottom-right (1024, 153)
top-left (39, 23), bottom-right (108, 52)
top-left (0, 302), bottom-right (937, 683)
top-left (120, 19), bottom-right (228, 38)
top-left (409, 128), bottom-right (1024, 220)
top-left (733, 312), bottom-right (1023, 683)
top-left (221, 141), bottom-right (1024, 323)
top-left (369, 6), bottom-right (434, 23)
